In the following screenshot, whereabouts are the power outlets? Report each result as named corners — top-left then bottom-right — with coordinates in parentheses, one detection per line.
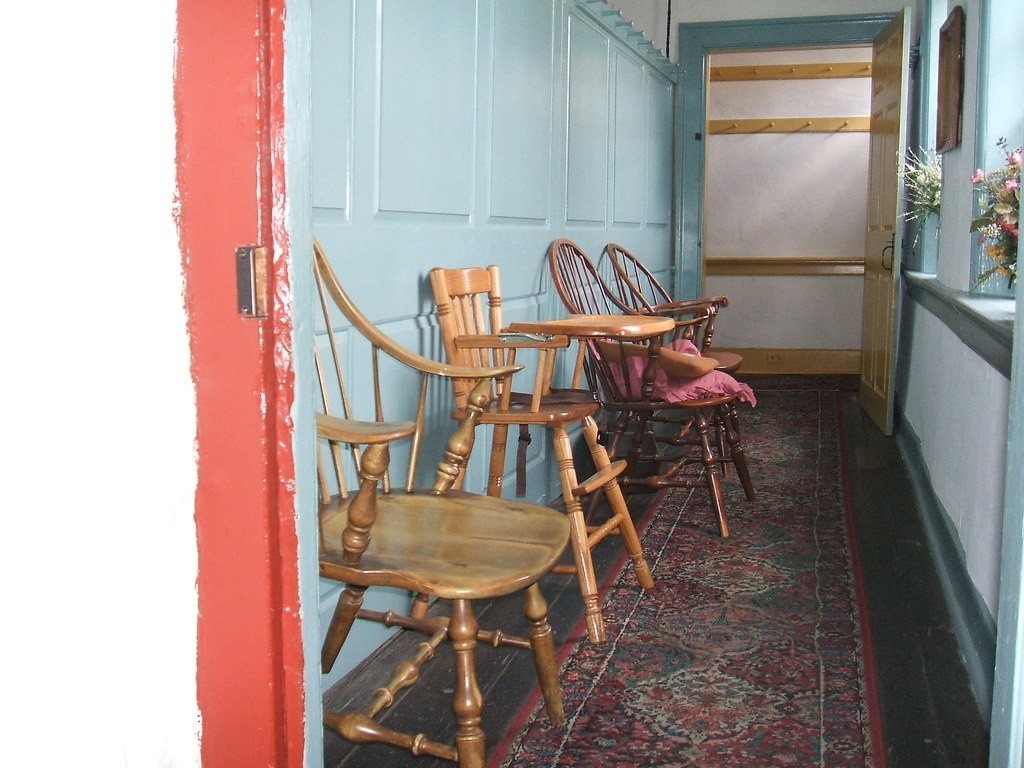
top-left (767, 353), bottom-right (784, 363)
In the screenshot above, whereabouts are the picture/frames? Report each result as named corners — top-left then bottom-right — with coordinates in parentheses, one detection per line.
top-left (936, 6), bottom-right (965, 154)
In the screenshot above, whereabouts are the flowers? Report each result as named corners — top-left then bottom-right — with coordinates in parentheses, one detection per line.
top-left (894, 140), bottom-right (942, 250)
top-left (964, 136), bottom-right (1024, 298)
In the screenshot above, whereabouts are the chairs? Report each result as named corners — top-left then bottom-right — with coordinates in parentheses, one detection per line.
top-left (409, 264), bottom-right (653, 647)
top-left (549, 240), bottom-right (757, 540)
top-left (312, 233), bottom-right (569, 768)
top-left (606, 243), bottom-right (743, 479)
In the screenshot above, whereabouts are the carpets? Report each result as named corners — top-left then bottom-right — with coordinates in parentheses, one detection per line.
top-left (486, 389), bottom-right (887, 768)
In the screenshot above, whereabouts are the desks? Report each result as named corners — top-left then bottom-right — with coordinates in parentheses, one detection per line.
top-left (506, 313), bottom-right (677, 341)
